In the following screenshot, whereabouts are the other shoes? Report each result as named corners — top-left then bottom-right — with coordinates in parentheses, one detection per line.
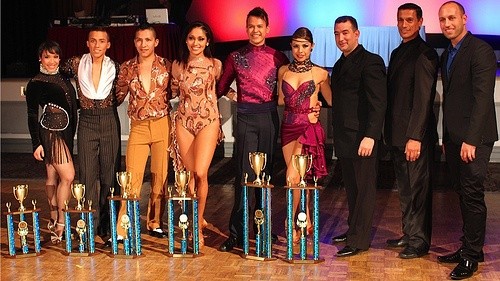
top-left (189, 219), bottom-right (207, 251)
top-left (285, 218), bottom-right (297, 245)
top-left (149, 228), bottom-right (167, 237)
top-left (306, 225), bottom-right (313, 235)
top-left (106, 235), bottom-right (124, 246)
top-left (94, 234), bottom-right (111, 247)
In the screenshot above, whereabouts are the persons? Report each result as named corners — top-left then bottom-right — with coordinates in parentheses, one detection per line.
top-left (168, 21), bottom-right (237, 250)
top-left (217, 6), bottom-right (322, 251)
top-left (437, 1), bottom-right (499, 279)
top-left (59, 24), bottom-right (124, 236)
top-left (386, 3), bottom-right (440, 259)
top-left (25, 39), bottom-right (80, 243)
top-left (277, 27), bottom-right (332, 246)
top-left (330, 16), bottom-right (388, 257)
top-left (104, 24), bottom-right (180, 249)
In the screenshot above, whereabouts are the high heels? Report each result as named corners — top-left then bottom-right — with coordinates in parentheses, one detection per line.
top-left (51, 221), bottom-right (65, 244)
top-left (48, 220), bottom-right (77, 241)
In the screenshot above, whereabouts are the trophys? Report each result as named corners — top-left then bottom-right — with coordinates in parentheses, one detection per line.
top-left (12, 184), bottom-right (29, 211)
top-left (249, 152), bottom-right (267, 184)
top-left (292, 154), bottom-right (313, 187)
top-left (116, 171), bottom-right (132, 198)
top-left (71, 184), bottom-right (86, 210)
top-left (174, 170), bottom-right (190, 197)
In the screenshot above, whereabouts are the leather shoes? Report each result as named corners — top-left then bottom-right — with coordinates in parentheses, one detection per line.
top-left (400, 246), bottom-right (429, 259)
top-left (270, 234), bottom-right (278, 241)
top-left (437, 247), bottom-right (484, 262)
top-left (386, 235), bottom-right (407, 246)
top-left (449, 257), bottom-right (479, 279)
top-left (219, 235), bottom-right (241, 252)
top-left (334, 245), bottom-right (359, 257)
top-left (332, 233), bottom-right (349, 242)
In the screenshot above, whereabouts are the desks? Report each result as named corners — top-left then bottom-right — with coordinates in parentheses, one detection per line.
top-left (48, 23), bottom-right (213, 65)
top-left (309, 26), bottom-right (426, 69)
